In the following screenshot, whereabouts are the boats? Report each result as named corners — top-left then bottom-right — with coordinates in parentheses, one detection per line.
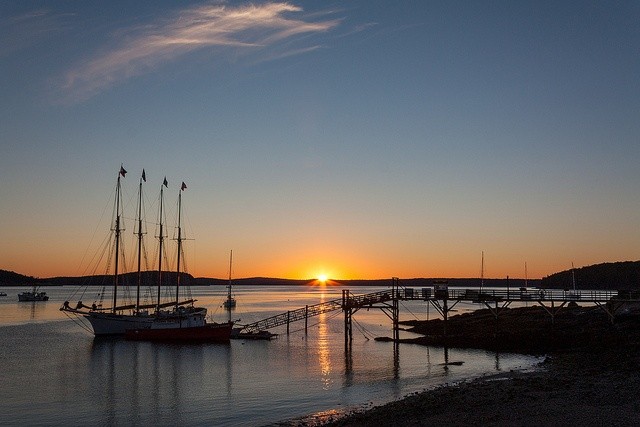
top-left (124, 309), bottom-right (241, 342)
top-left (0, 292), bottom-right (7, 296)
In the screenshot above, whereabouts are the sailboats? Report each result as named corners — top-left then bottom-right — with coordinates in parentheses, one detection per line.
top-left (18, 277), bottom-right (50, 302)
top-left (507, 260), bottom-right (544, 296)
top-left (566, 262), bottom-right (583, 300)
top-left (224, 249), bottom-right (236, 309)
top-left (458, 250), bottom-right (505, 301)
top-left (60, 168), bottom-right (207, 335)
